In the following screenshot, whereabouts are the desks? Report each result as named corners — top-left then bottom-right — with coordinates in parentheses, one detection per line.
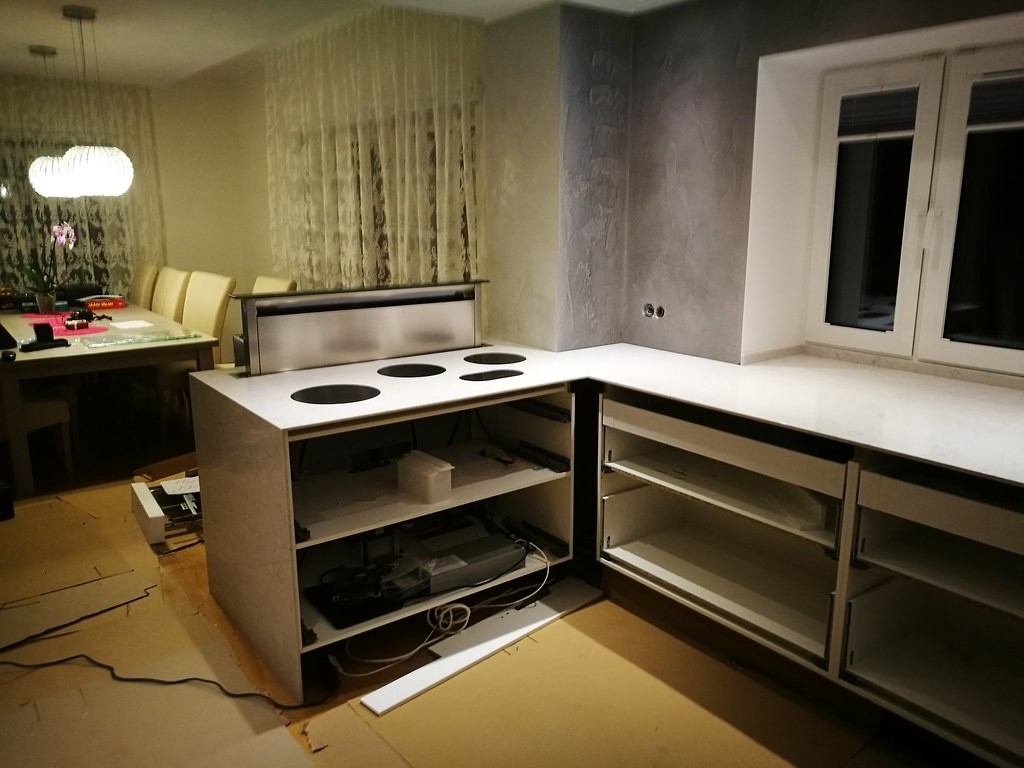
top-left (0, 301), bottom-right (220, 500)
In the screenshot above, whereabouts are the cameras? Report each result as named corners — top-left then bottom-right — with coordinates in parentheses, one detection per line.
top-left (71, 309), bottom-right (93, 322)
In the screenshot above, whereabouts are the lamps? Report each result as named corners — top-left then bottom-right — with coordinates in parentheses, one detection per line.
top-left (62, 5), bottom-right (134, 196)
top-left (28, 45), bottom-right (81, 198)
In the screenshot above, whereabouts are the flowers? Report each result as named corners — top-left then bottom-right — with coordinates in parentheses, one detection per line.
top-left (14, 204), bottom-right (85, 292)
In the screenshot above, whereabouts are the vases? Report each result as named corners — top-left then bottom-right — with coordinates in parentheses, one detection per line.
top-left (35, 290), bottom-right (56, 313)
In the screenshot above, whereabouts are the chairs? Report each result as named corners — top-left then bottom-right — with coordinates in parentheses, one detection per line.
top-left (214, 276), bottom-right (297, 370)
top-left (151, 266), bottom-right (192, 324)
top-left (128, 263), bottom-right (158, 309)
top-left (182, 270), bottom-right (236, 343)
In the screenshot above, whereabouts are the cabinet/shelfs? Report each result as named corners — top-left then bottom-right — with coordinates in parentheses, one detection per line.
top-left (188, 344), bottom-right (574, 713)
top-left (564, 344), bottom-right (1024, 768)
